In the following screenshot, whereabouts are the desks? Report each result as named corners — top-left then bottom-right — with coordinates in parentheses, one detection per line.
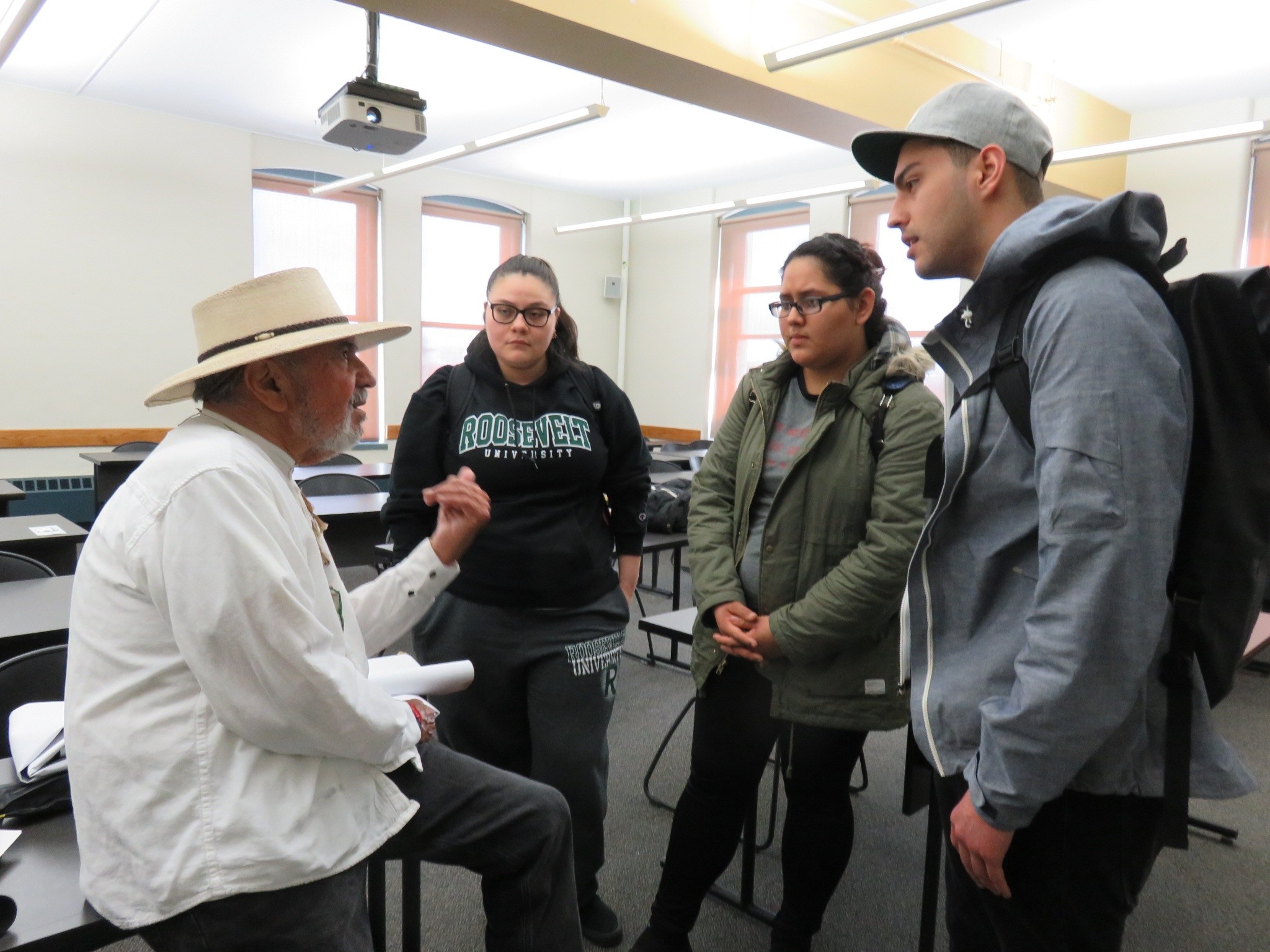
top-left (0, 439), bottom-right (868, 952)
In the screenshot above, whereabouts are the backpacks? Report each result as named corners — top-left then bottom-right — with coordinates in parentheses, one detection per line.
top-left (950, 233), bottom-right (1270, 850)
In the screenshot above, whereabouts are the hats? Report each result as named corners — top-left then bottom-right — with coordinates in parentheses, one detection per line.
top-left (145, 268), bottom-right (412, 409)
top-left (851, 82), bottom-right (1054, 181)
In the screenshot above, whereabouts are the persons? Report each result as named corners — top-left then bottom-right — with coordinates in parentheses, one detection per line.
top-left (597, 234), bottom-right (947, 952)
top-left (62, 264), bottom-right (588, 952)
top-left (383, 256), bottom-right (653, 942)
top-left (850, 78), bottom-right (1260, 952)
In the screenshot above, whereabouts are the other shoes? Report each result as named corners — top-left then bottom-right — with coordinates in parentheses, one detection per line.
top-left (768, 929), bottom-right (810, 952)
top-left (629, 924), bottom-right (692, 952)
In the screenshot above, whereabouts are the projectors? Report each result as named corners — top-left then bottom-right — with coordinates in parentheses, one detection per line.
top-left (319, 92), bottom-right (426, 156)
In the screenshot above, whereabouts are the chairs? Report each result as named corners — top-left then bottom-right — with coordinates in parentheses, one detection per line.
top-left (0, 643), bottom-right (67, 759)
top-left (297, 473), bottom-right (381, 495)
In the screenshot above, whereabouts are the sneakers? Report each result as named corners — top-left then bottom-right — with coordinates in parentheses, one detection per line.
top-left (577, 891), bottom-right (624, 948)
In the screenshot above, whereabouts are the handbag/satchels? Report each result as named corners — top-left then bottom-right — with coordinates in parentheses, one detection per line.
top-left (646, 479), bottom-right (691, 534)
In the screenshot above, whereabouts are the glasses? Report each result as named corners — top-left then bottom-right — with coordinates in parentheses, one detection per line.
top-left (486, 299), bottom-right (556, 327)
top-left (769, 291), bottom-right (851, 318)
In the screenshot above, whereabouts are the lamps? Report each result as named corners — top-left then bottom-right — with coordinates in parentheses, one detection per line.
top-left (308, 0), bottom-right (1270, 233)
